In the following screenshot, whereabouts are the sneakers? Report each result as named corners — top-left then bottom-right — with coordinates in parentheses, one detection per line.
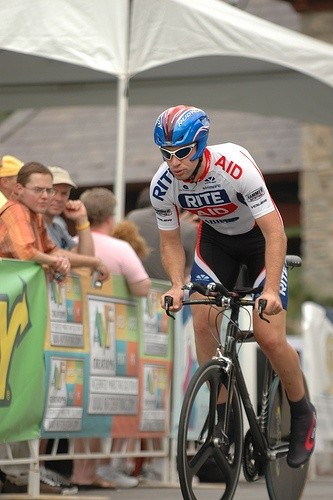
top-left (286, 405), bottom-right (317, 468)
top-left (207, 426), bottom-right (237, 461)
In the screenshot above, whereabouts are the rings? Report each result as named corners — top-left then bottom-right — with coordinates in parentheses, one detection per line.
top-left (64, 265), bottom-right (68, 268)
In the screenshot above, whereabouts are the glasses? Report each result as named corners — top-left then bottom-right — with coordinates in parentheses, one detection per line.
top-left (158, 141), bottom-right (198, 161)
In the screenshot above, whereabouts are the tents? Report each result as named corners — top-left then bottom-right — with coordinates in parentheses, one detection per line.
top-left (0, 1), bottom-right (333, 220)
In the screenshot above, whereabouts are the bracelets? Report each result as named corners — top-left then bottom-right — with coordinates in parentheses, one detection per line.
top-left (75, 221), bottom-right (91, 231)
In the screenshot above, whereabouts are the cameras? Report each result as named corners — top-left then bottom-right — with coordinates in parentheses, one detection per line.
top-left (90, 269), bottom-right (103, 289)
top-left (54, 270), bottom-right (64, 281)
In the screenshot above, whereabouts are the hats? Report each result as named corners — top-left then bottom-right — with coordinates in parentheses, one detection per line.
top-left (0, 155), bottom-right (25, 177)
top-left (47, 166), bottom-right (78, 189)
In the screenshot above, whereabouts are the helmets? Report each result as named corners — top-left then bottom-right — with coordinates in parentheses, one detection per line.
top-left (153, 105), bottom-right (211, 161)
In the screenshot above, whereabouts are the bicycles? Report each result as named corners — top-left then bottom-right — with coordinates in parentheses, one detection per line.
top-left (163, 254), bottom-right (311, 500)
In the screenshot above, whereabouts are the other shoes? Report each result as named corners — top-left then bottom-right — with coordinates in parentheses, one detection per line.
top-left (69, 478), bottom-right (119, 490)
top-left (8, 469), bottom-right (63, 494)
top-left (96, 465), bottom-right (138, 488)
top-left (142, 462), bottom-right (199, 488)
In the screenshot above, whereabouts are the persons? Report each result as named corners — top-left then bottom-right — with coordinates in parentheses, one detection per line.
top-left (147, 105), bottom-right (317, 468)
top-left (109, 218), bottom-right (151, 479)
top-left (0, 155), bottom-right (24, 209)
top-left (42, 165), bottom-right (117, 490)
top-left (70, 186), bottom-right (150, 487)
top-left (0, 162), bottom-right (109, 496)
